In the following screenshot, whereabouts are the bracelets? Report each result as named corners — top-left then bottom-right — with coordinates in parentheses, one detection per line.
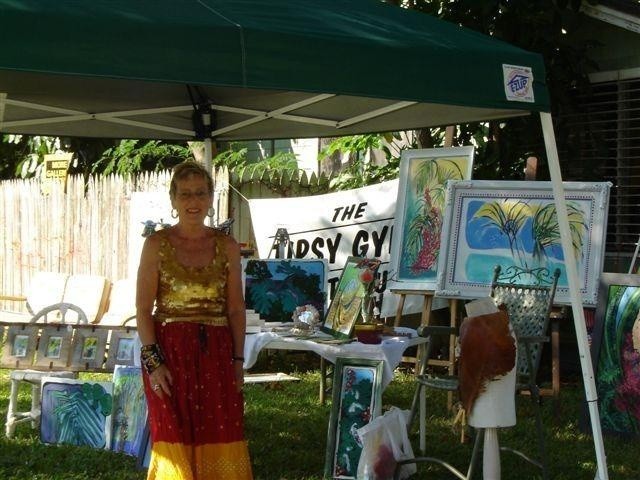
top-left (232, 356), bottom-right (245, 363)
top-left (139, 344), bottom-right (165, 375)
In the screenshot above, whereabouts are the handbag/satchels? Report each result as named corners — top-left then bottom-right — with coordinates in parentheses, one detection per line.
top-left (355, 406), bottom-right (417, 480)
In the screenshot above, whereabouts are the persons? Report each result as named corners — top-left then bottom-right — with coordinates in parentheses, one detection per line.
top-left (134, 161), bottom-right (254, 480)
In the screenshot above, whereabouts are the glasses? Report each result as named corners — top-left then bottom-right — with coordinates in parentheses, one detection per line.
top-left (177, 191), bottom-right (207, 199)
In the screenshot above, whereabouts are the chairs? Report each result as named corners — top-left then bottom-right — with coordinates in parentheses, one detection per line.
top-left (0, 272), bottom-right (144, 326)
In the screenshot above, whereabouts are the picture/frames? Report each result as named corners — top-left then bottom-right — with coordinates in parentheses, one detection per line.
top-left (0, 326), bottom-right (151, 458)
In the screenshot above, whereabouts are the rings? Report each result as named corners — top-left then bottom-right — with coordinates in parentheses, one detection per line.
top-left (151, 384), bottom-right (160, 391)
